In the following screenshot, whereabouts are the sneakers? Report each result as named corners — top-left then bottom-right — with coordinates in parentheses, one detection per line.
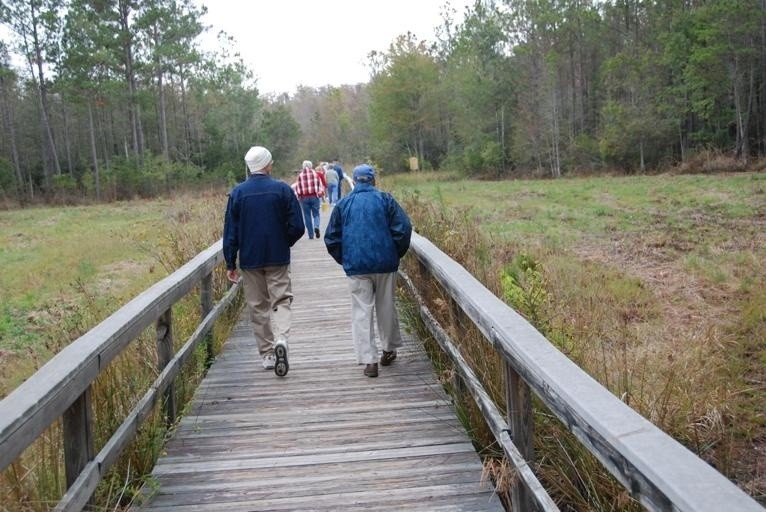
top-left (275, 340), bottom-right (289, 377)
top-left (262, 355), bottom-right (277, 370)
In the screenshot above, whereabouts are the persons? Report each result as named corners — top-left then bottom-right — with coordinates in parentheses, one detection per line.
top-left (296, 160), bottom-right (324, 239)
top-left (314, 158), bottom-right (344, 206)
top-left (324, 164), bottom-right (412, 377)
top-left (222, 146), bottom-right (305, 376)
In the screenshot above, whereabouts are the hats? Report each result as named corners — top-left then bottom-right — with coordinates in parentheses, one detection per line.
top-left (353, 164), bottom-right (376, 183)
top-left (244, 146), bottom-right (272, 172)
top-left (302, 160), bottom-right (312, 168)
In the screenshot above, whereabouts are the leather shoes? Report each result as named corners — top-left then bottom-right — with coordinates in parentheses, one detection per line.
top-left (315, 228), bottom-right (320, 239)
top-left (364, 362), bottom-right (379, 378)
top-left (380, 350), bottom-right (397, 366)
